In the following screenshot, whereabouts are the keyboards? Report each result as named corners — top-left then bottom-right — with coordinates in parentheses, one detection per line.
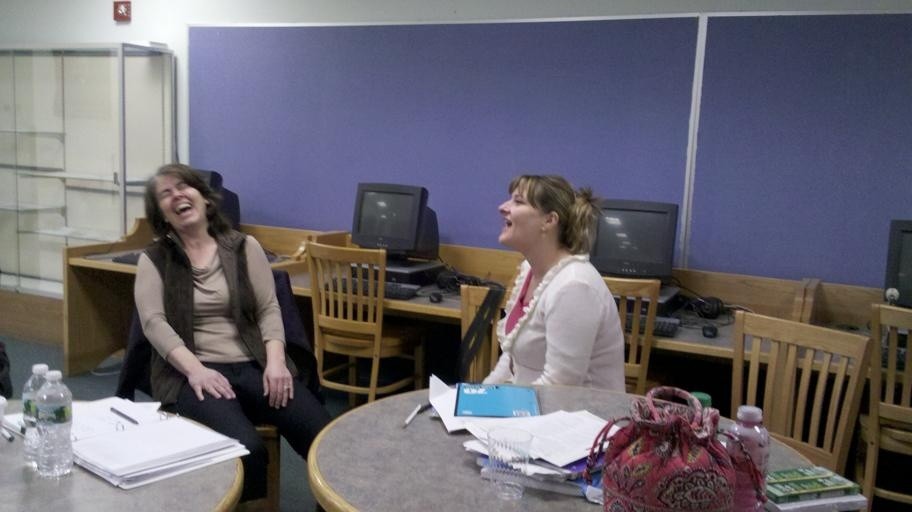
top-left (881, 345), bottom-right (905, 371)
top-left (617, 311), bottom-right (680, 337)
top-left (324, 277), bottom-right (420, 299)
top-left (112, 253), bottom-right (140, 264)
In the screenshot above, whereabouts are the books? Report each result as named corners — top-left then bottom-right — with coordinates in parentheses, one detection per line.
top-left (2, 393), bottom-right (251, 490)
top-left (764, 461), bottom-right (861, 504)
top-left (425, 372), bottom-right (623, 503)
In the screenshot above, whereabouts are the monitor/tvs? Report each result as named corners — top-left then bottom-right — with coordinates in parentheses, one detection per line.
top-left (192, 169), bottom-right (240, 230)
top-left (884, 220), bottom-right (912, 308)
top-left (590, 199), bottom-right (678, 279)
top-left (351, 183), bottom-right (439, 267)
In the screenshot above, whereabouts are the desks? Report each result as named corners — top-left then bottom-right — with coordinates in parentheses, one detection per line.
top-left (62, 216), bottom-right (912, 439)
top-left (0, 395), bottom-right (244, 512)
top-left (307, 383), bottom-right (816, 511)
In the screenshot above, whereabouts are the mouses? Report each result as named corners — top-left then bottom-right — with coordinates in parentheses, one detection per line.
top-left (704, 326), bottom-right (717, 338)
top-left (430, 292), bottom-right (441, 302)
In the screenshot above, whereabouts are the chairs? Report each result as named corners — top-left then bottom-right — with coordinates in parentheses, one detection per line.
top-left (730, 308), bottom-right (873, 477)
top-left (600, 275), bottom-right (664, 396)
top-left (853, 302), bottom-right (911, 512)
top-left (306, 240), bottom-right (425, 401)
top-left (459, 284), bottom-right (511, 383)
top-left (113, 269), bottom-right (324, 511)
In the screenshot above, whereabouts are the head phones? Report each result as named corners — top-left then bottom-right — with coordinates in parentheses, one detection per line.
top-left (688, 296), bottom-right (723, 318)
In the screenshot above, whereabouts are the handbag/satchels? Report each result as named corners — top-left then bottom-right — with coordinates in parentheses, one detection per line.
top-left (583, 383), bottom-right (770, 510)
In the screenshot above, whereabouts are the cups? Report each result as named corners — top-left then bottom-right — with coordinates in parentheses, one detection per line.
top-left (486, 424), bottom-right (532, 499)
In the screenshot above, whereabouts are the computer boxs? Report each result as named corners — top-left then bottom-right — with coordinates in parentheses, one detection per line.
top-left (613, 286), bottom-right (680, 316)
top-left (350, 260), bottom-right (444, 285)
top-left (886, 327), bottom-right (907, 349)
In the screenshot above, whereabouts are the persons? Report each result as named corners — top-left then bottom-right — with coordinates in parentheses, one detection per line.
top-left (481, 170), bottom-right (627, 393)
top-left (133, 162), bottom-right (336, 511)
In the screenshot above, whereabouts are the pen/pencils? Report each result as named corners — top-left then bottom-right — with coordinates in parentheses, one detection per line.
top-left (110, 407), bottom-right (138, 425)
top-left (417, 403), bottom-right (432, 415)
top-left (0, 425), bottom-right (14, 441)
top-left (429, 414), bottom-right (441, 421)
top-left (3, 419), bottom-right (26, 434)
top-left (401, 403), bottom-right (423, 429)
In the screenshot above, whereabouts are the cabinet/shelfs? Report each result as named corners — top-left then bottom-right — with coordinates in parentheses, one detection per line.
top-left (0, 41), bottom-right (174, 349)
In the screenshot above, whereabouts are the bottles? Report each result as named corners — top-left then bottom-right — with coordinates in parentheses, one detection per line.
top-left (725, 404), bottom-right (769, 511)
top-left (22, 361), bottom-right (50, 477)
top-left (36, 371), bottom-right (75, 476)
